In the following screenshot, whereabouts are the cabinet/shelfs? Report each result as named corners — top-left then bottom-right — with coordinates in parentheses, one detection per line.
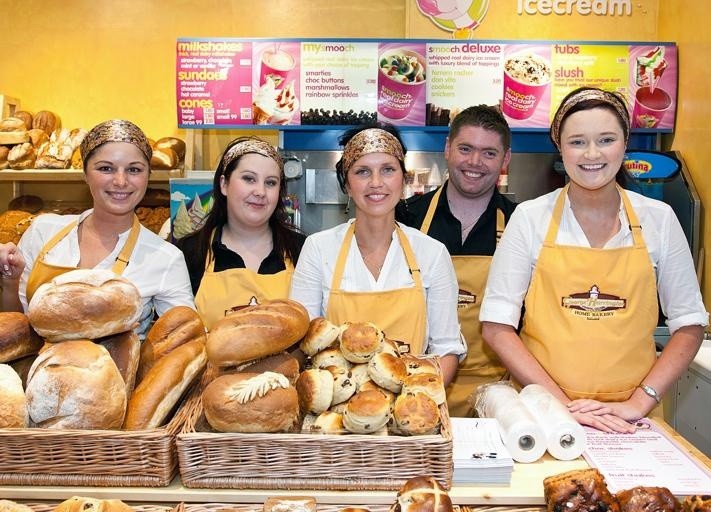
top-left (662, 340), bottom-right (710, 459)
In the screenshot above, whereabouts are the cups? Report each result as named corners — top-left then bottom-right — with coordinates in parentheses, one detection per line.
top-left (630, 86), bottom-right (672, 128)
top-left (500, 59), bottom-right (551, 120)
top-left (265, 90), bottom-right (298, 125)
top-left (378, 49), bottom-right (427, 124)
top-left (499, 174), bottom-right (510, 194)
top-left (260, 47), bottom-right (297, 90)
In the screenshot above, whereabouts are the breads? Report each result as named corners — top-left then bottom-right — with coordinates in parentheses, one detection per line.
top-left (1, 194), bottom-right (457, 511)
top-left (541, 468), bottom-right (711, 511)
top-left (0, 110), bottom-right (185, 170)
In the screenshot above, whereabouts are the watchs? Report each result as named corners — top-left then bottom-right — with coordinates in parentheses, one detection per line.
top-left (637, 382), bottom-right (662, 403)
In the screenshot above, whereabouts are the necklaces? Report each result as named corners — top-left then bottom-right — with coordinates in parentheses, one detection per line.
top-left (462, 218), bottom-right (480, 233)
top-left (362, 256), bottom-right (384, 272)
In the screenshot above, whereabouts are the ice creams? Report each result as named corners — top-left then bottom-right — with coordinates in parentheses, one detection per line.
top-left (636, 45), bottom-right (668, 95)
top-left (254, 76), bottom-right (301, 126)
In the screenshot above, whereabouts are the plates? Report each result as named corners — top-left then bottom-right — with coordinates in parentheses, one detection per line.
top-left (7, 195), bottom-right (42, 214)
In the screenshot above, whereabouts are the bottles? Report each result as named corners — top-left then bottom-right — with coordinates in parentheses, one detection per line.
top-left (282, 193), bottom-right (301, 228)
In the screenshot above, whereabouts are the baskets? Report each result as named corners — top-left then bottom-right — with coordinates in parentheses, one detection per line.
top-left (175, 354), bottom-right (453, 491)
top-left (0, 359), bottom-right (205, 488)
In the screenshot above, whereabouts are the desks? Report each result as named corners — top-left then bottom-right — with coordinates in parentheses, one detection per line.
top-left (0, 414), bottom-right (711, 505)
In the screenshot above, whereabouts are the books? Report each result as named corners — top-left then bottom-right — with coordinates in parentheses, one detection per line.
top-left (449, 416), bottom-right (515, 483)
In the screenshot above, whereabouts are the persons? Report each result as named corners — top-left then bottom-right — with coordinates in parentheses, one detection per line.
top-left (479, 86), bottom-right (711, 432)
top-left (395, 103), bottom-right (526, 415)
top-left (287, 124), bottom-right (469, 390)
top-left (153, 136), bottom-right (308, 369)
top-left (0, 119), bottom-right (209, 348)
